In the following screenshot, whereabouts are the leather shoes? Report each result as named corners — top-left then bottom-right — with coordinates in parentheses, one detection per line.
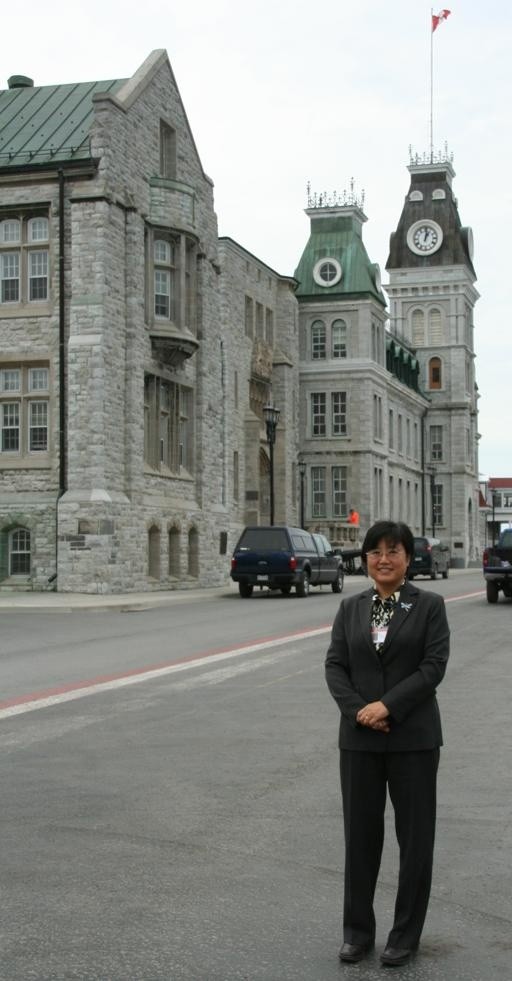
top-left (339, 943), bottom-right (366, 961)
top-left (381, 946), bottom-right (412, 965)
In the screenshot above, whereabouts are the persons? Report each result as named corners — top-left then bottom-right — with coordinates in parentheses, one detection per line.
top-left (325, 520), bottom-right (451, 968)
top-left (347, 509), bottom-right (359, 524)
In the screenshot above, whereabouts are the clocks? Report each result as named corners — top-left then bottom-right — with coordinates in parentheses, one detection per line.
top-left (407, 219), bottom-right (443, 257)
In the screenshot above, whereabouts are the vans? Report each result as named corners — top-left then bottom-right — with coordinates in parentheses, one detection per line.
top-left (229, 526), bottom-right (344, 596)
top-left (411, 537), bottom-right (452, 579)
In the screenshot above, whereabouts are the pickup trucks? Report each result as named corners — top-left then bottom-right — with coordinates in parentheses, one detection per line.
top-left (483, 529), bottom-right (511, 603)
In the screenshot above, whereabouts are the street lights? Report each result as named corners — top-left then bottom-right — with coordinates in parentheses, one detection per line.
top-left (264, 404), bottom-right (281, 527)
top-left (490, 489), bottom-right (497, 548)
